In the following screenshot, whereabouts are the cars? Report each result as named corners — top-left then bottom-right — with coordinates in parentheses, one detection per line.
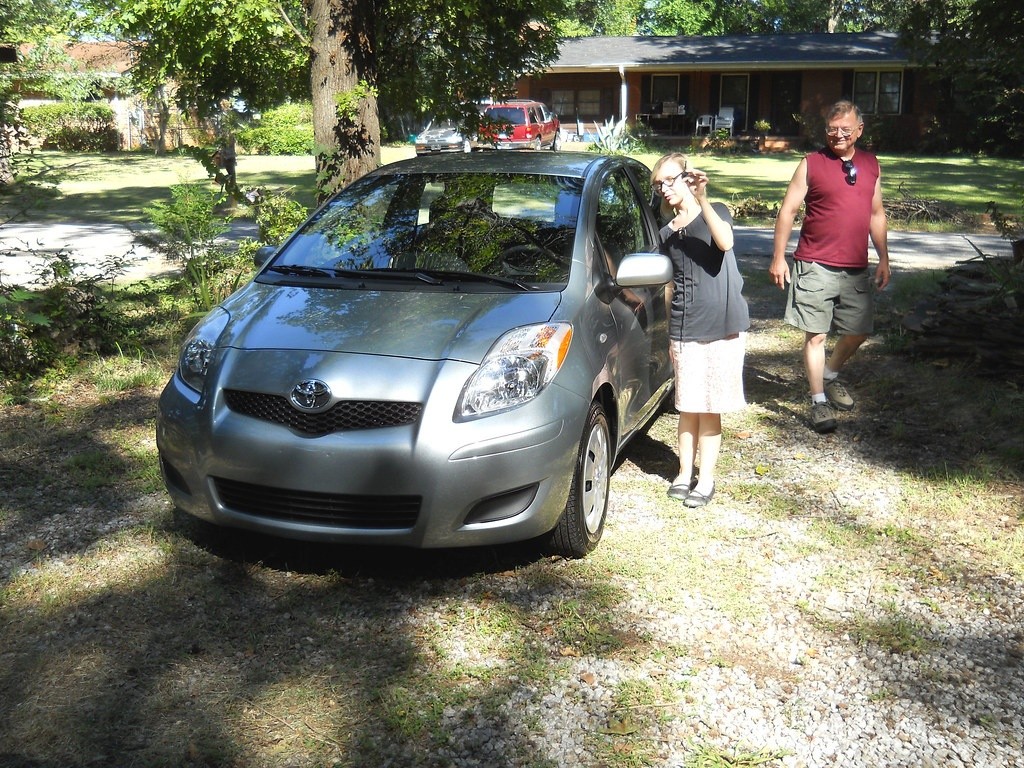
top-left (154, 150), bottom-right (678, 559)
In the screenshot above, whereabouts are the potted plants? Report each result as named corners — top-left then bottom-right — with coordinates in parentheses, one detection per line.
top-left (753, 119), bottom-right (771, 138)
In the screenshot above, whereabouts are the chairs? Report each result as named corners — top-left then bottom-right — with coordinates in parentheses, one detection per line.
top-left (714, 107), bottom-right (734, 138)
top-left (694, 115), bottom-right (713, 136)
top-left (414, 195), bottom-right (463, 256)
top-left (553, 186), bottom-right (600, 226)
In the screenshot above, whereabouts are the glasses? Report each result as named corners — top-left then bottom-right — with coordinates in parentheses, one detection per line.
top-left (651, 171), bottom-right (684, 191)
top-left (844, 160), bottom-right (856, 184)
top-left (825, 122), bottom-right (860, 137)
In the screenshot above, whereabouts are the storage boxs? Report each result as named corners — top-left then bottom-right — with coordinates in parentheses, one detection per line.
top-left (583, 133), bottom-right (599, 142)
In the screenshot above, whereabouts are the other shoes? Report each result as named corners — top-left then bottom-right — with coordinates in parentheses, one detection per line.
top-left (667, 473), bottom-right (715, 508)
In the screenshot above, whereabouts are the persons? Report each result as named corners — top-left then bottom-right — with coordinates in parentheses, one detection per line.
top-left (649, 152), bottom-right (750, 509)
top-left (766, 101), bottom-right (891, 432)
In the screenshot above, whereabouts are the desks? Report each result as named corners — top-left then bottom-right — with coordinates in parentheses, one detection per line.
top-left (635, 114), bottom-right (685, 137)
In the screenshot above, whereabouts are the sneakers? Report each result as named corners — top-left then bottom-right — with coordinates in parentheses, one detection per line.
top-left (810, 396), bottom-right (837, 434)
top-left (823, 377), bottom-right (854, 411)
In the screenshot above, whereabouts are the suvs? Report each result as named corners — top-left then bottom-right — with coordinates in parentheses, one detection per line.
top-left (479, 99), bottom-right (561, 152)
top-left (414, 101), bottom-right (490, 156)
